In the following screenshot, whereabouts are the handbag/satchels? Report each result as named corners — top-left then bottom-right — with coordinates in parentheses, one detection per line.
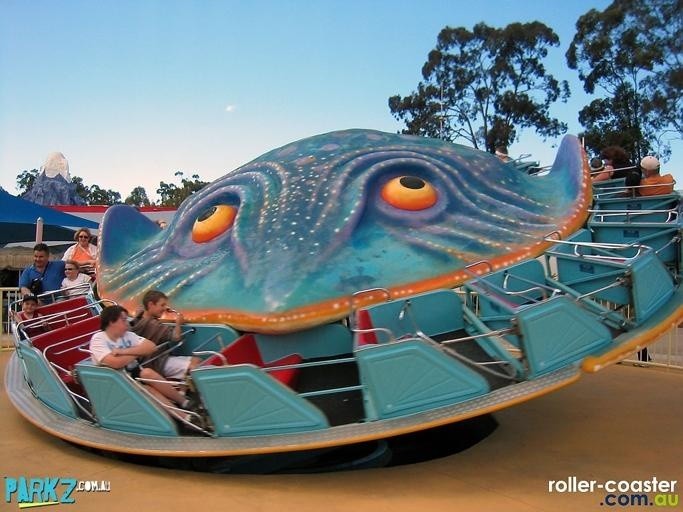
top-left (31, 262), bottom-right (50, 294)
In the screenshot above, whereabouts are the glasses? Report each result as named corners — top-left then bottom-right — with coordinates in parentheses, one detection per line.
top-left (65, 267), bottom-right (76, 271)
top-left (78, 235), bottom-right (89, 239)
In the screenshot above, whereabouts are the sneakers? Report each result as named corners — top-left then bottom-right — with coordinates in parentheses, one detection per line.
top-left (182, 396), bottom-right (207, 431)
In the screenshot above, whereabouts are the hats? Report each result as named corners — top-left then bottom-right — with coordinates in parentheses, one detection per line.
top-left (23, 292), bottom-right (38, 304)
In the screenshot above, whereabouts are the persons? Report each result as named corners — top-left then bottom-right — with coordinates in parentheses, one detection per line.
top-left (492, 145), bottom-right (515, 165)
top-left (7, 292), bottom-right (52, 339)
top-left (87, 305), bottom-right (207, 430)
top-left (54, 258), bottom-right (92, 306)
top-left (59, 226), bottom-right (97, 271)
top-left (588, 146), bottom-right (673, 198)
top-left (18, 242), bottom-right (97, 313)
top-left (628, 346), bottom-right (653, 367)
top-left (126, 289), bottom-right (207, 413)
